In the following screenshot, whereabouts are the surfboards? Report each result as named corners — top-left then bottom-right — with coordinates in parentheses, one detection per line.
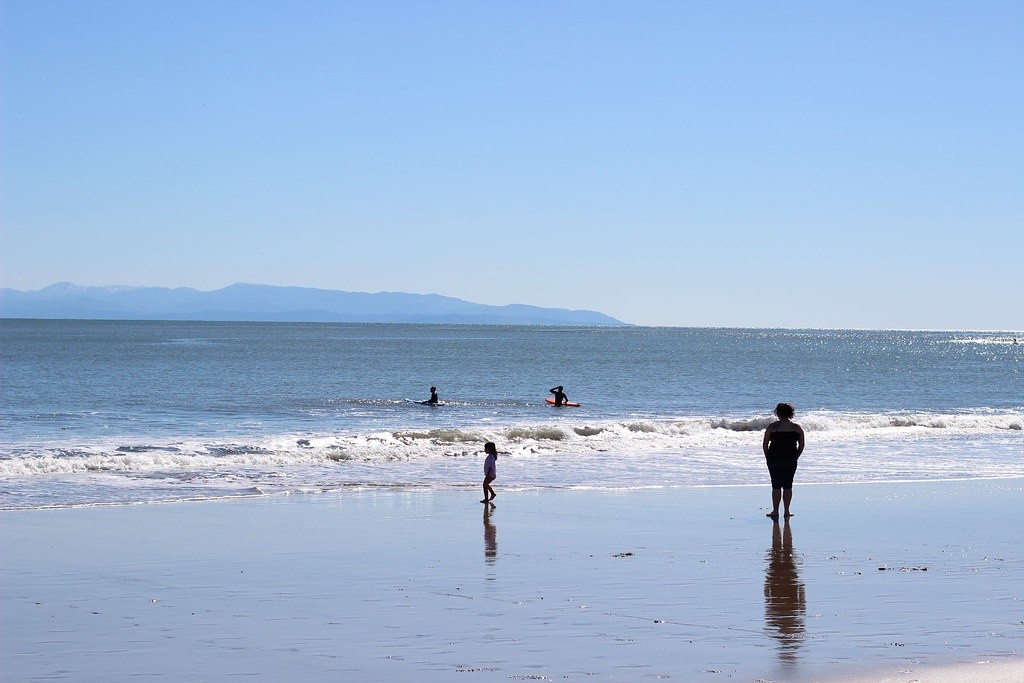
top-left (545, 397), bottom-right (581, 406)
top-left (404, 398), bottom-right (445, 406)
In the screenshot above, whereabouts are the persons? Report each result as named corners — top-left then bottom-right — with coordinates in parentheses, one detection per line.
top-left (550, 386), bottom-right (568, 407)
top-left (422, 387), bottom-right (438, 404)
top-left (762, 403), bottom-right (804, 517)
top-left (480, 442), bottom-right (497, 502)
